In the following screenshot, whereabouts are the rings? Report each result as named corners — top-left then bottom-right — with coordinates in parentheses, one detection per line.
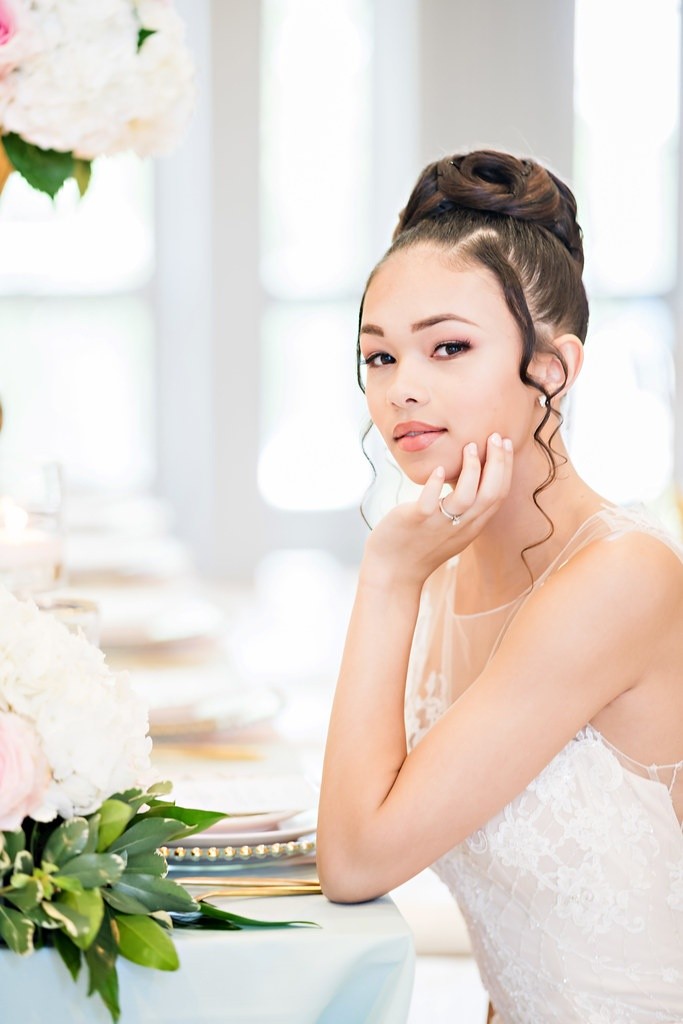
top-left (439, 497), bottom-right (458, 522)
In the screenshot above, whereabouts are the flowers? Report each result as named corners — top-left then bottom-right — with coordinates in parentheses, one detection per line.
top-left (0, 583), bottom-right (324, 1024)
top-left (0, 0), bottom-right (200, 211)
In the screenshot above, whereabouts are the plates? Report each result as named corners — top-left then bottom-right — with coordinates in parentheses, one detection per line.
top-left (156, 811), bottom-right (318, 871)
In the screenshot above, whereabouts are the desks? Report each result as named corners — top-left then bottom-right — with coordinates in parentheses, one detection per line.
top-left (0, 587), bottom-right (417, 1024)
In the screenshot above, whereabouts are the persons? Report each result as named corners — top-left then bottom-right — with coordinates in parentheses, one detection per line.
top-left (316, 150), bottom-right (683, 1024)
